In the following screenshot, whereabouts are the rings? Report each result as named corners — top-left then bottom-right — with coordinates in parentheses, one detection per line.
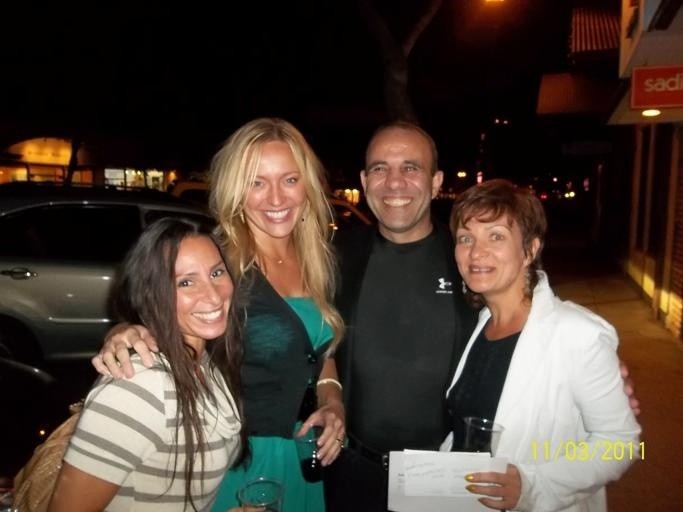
top-left (336, 438), bottom-right (343, 448)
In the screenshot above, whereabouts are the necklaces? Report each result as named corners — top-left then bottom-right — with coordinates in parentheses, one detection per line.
top-left (256, 242), bottom-right (296, 266)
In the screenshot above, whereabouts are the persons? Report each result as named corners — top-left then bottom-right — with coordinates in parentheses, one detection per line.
top-left (440, 177), bottom-right (643, 512)
top-left (44, 216), bottom-right (270, 511)
top-left (302, 119), bottom-right (640, 512)
top-left (88, 116), bottom-right (347, 512)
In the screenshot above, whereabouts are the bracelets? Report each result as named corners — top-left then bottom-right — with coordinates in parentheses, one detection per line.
top-left (316, 378), bottom-right (342, 391)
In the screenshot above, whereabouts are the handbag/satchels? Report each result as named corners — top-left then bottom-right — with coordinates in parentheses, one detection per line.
top-left (13, 398), bottom-right (86, 512)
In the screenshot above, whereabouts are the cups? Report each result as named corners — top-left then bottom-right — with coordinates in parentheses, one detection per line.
top-left (462, 416), bottom-right (502, 455)
top-left (238, 479), bottom-right (283, 512)
top-left (295, 428), bottom-right (325, 483)
